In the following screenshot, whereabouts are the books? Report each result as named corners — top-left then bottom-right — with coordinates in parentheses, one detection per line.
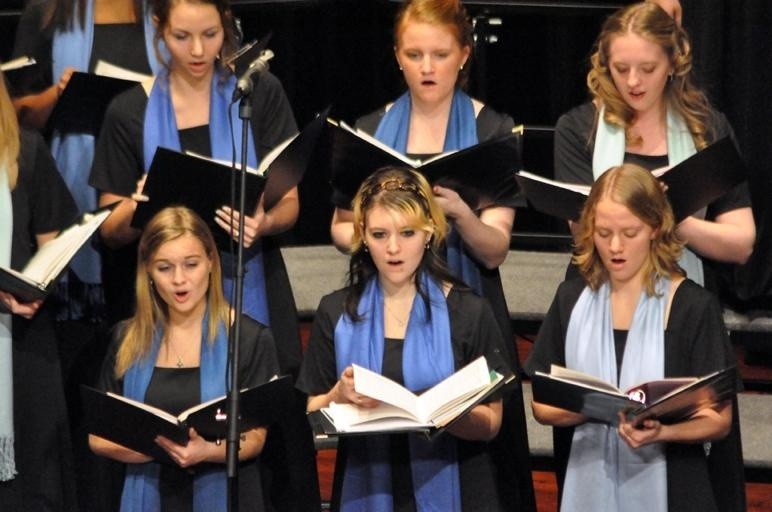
top-left (44, 58), bottom-right (158, 136)
top-left (314, 113), bottom-right (521, 221)
top-left (128, 129), bottom-right (307, 254)
top-left (504, 133), bottom-right (749, 228)
top-left (306, 354), bottom-right (517, 447)
top-left (78, 374), bottom-right (296, 468)
top-left (528, 362), bottom-right (742, 428)
top-left (0, 201), bottom-right (123, 313)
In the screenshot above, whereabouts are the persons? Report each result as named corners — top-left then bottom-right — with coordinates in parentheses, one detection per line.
top-left (9, 1), bottom-right (173, 328)
top-left (87, 0), bottom-right (323, 511)
top-left (0, 71), bottom-right (103, 509)
top-left (520, 163), bottom-right (745, 512)
top-left (520, 2), bottom-right (758, 510)
top-left (73, 204), bottom-right (286, 511)
top-left (296, 165), bottom-right (519, 511)
top-left (314, 0), bottom-right (540, 511)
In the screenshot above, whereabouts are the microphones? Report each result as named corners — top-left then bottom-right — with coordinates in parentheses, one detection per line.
top-left (233, 49), bottom-right (275, 104)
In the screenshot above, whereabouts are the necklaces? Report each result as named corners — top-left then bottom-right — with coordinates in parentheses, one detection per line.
top-left (382, 300), bottom-right (410, 328)
top-left (167, 309), bottom-right (204, 369)
